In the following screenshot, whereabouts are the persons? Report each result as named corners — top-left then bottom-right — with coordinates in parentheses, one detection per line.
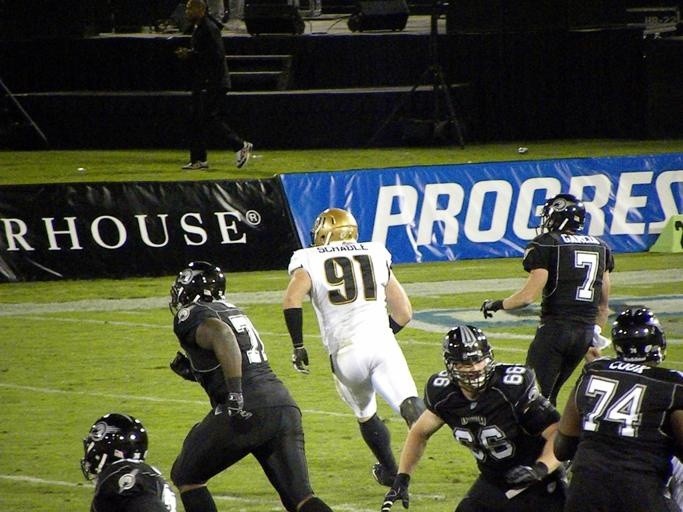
top-left (282, 208), bottom-right (430, 488)
top-left (168, 259), bottom-right (337, 512)
top-left (175, 0), bottom-right (253, 170)
top-left (81, 413), bottom-right (178, 512)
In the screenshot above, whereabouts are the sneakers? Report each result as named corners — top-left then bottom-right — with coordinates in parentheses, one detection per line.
top-left (180, 159), bottom-right (210, 171)
top-left (369, 463), bottom-right (401, 491)
top-left (234, 137), bottom-right (255, 168)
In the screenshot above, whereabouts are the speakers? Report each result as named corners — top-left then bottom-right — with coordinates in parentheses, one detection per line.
top-left (244, 4), bottom-right (305, 36)
top-left (347, 1), bottom-right (411, 32)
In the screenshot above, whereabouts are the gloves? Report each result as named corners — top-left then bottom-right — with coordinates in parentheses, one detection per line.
top-left (380, 472), bottom-right (410, 512)
top-left (168, 351), bottom-right (198, 384)
top-left (222, 392), bottom-right (257, 424)
top-left (290, 343), bottom-right (312, 376)
top-left (502, 461), bottom-right (550, 489)
top-left (480, 299), bottom-right (505, 319)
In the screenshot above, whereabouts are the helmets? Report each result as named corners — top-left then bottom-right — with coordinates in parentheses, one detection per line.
top-left (609, 305), bottom-right (668, 367)
top-left (79, 412), bottom-right (150, 483)
top-left (308, 206), bottom-right (362, 248)
top-left (166, 259), bottom-right (229, 310)
top-left (441, 323), bottom-right (497, 396)
top-left (535, 189), bottom-right (587, 234)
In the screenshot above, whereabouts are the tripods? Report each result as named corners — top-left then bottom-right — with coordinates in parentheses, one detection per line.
top-left (364, 8), bottom-right (467, 150)
